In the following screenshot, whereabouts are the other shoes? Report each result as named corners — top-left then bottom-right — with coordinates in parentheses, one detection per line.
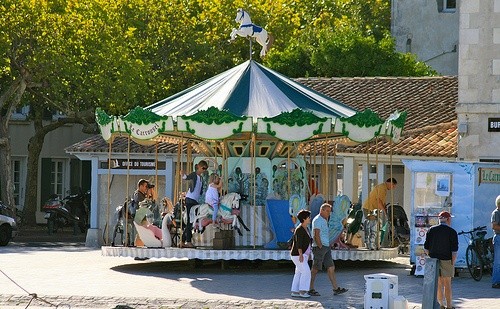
top-left (446, 306), bottom-right (455, 309)
top-left (179, 241), bottom-right (186, 247)
top-left (299, 293), bottom-right (310, 297)
top-left (291, 292), bottom-right (299, 296)
top-left (492, 283), bottom-right (500, 289)
top-left (186, 242), bottom-right (194, 248)
top-left (440, 306), bottom-right (445, 309)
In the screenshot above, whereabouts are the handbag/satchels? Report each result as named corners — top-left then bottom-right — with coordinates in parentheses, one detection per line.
top-left (128, 202), bottom-right (138, 216)
top-left (288, 238), bottom-right (293, 246)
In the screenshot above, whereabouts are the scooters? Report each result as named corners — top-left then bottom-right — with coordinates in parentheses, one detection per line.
top-left (43, 191), bottom-right (91, 233)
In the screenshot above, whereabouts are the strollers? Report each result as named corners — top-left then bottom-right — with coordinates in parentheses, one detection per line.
top-left (385, 202), bottom-right (411, 254)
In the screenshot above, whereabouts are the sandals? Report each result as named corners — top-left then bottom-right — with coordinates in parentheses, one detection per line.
top-left (308, 289), bottom-right (320, 296)
top-left (333, 287), bottom-right (348, 295)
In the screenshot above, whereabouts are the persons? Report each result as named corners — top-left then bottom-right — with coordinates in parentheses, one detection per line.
top-left (307, 203), bottom-right (349, 297)
top-left (423, 211), bottom-right (459, 309)
top-left (490, 195), bottom-right (500, 288)
top-left (179, 160), bottom-right (208, 247)
top-left (362, 177), bottom-right (398, 220)
top-left (133, 179), bottom-right (155, 204)
top-left (205, 173), bottom-right (222, 229)
top-left (290, 210), bottom-right (313, 298)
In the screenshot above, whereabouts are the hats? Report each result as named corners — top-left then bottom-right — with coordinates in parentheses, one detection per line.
top-left (439, 211), bottom-right (454, 218)
top-left (147, 184), bottom-right (154, 189)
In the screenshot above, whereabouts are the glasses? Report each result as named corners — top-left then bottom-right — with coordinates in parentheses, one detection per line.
top-left (203, 167), bottom-right (208, 170)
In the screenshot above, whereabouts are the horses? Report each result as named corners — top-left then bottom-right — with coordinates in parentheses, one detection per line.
top-left (111, 192), bottom-right (363, 247)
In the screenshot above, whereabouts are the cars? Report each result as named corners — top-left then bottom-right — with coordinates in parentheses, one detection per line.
top-left (0, 214), bottom-right (17, 247)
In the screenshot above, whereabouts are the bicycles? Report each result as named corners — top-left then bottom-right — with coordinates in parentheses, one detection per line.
top-left (457, 225), bottom-right (495, 281)
